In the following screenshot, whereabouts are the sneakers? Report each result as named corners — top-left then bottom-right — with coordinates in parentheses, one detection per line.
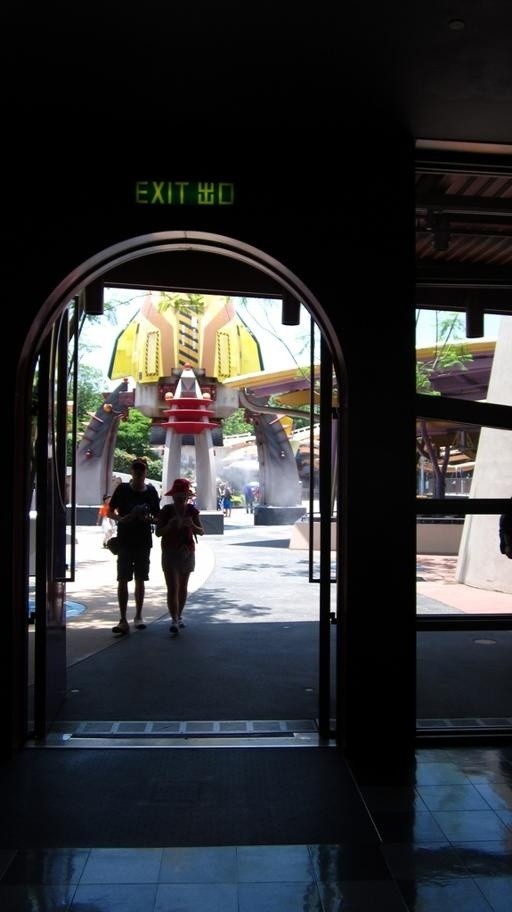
top-left (170, 619), bottom-right (179, 632)
top-left (179, 617), bottom-right (185, 628)
top-left (112, 618), bottom-right (129, 632)
top-left (134, 616), bottom-right (146, 629)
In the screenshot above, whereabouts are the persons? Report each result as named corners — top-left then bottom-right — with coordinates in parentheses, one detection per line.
top-left (217, 482), bottom-right (255, 517)
top-left (155, 478), bottom-right (204, 633)
top-left (106, 458), bottom-right (163, 632)
top-left (96, 495), bottom-right (119, 546)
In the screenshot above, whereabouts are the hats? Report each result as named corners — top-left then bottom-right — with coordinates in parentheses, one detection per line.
top-left (131, 460), bottom-right (147, 466)
top-left (164, 479), bottom-right (194, 496)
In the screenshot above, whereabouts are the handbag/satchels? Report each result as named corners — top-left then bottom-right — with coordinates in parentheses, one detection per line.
top-left (107, 537), bottom-right (118, 556)
